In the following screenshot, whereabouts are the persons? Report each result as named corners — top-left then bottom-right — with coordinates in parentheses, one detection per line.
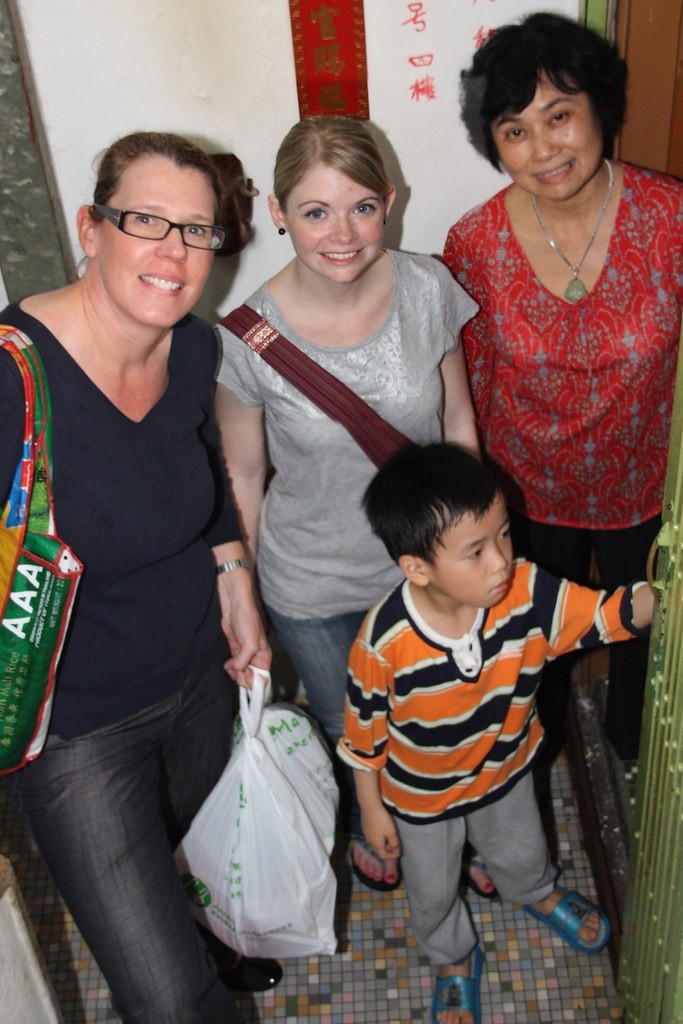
top-left (0, 132), bottom-right (284, 1023)
top-left (334, 442), bottom-right (656, 1024)
top-left (209, 117), bottom-right (499, 898)
top-left (441, 12), bottom-right (683, 763)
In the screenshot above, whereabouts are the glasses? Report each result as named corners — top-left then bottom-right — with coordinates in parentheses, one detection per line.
top-left (92, 198), bottom-right (226, 251)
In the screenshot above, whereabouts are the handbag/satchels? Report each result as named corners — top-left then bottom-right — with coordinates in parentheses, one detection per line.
top-left (0, 323), bottom-right (85, 778)
top-left (170, 663), bottom-right (339, 961)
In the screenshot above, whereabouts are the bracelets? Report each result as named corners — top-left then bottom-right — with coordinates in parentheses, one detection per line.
top-left (215, 559), bottom-right (252, 574)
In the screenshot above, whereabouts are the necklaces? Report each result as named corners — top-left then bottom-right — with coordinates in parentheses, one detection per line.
top-left (531, 157), bottom-right (613, 302)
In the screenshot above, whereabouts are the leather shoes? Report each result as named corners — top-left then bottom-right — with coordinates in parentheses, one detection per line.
top-left (216, 955), bottom-right (282, 994)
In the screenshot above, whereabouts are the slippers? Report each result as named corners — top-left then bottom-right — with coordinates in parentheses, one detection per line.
top-left (462, 847), bottom-right (499, 899)
top-left (428, 943), bottom-right (482, 1023)
top-left (523, 886), bottom-right (612, 953)
top-left (349, 835), bottom-right (403, 892)
